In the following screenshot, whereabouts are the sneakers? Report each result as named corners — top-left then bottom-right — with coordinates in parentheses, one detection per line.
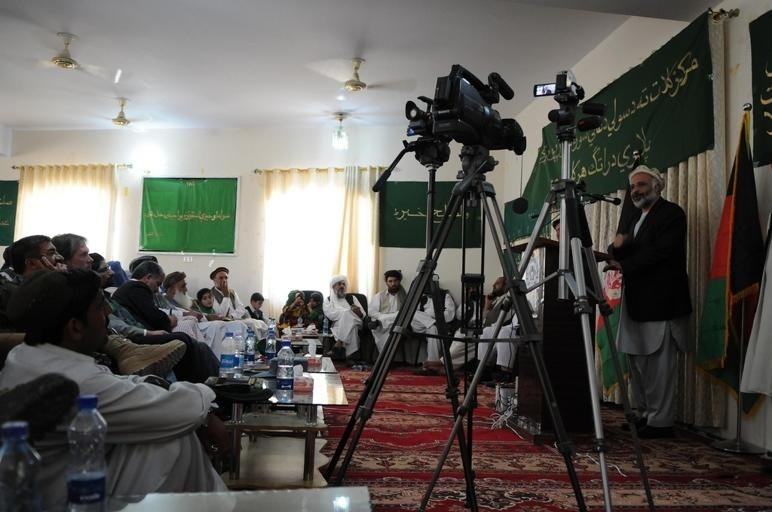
top-left (0, 370), bottom-right (81, 454)
top-left (621, 416), bottom-right (675, 440)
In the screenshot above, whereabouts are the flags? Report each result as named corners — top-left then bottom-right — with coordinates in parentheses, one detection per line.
top-left (741, 207), bottom-right (772, 397)
top-left (596, 269), bottom-right (622, 395)
top-left (697, 109), bottom-right (766, 419)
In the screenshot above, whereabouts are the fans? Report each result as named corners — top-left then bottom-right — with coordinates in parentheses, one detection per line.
top-left (68, 96), bottom-right (147, 128)
top-left (307, 107), bottom-right (372, 127)
top-left (0, 28), bottom-right (150, 100)
top-left (297, 54), bottom-right (420, 99)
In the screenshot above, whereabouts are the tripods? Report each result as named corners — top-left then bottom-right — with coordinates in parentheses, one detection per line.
top-left (419, 142), bottom-right (655, 512)
top-left (334, 156), bottom-right (587, 512)
top-left (324, 168), bottom-right (478, 512)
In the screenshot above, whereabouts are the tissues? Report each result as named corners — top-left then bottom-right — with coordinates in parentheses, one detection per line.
top-left (306, 340), bottom-right (323, 369)
top-left (293, 364), bottom-right (313, 391)
top-left (306, 324), bottom-right (318, 336)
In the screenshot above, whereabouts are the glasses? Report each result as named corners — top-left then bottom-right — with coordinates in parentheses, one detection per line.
top-left (99, 264), bottom-right (112, 271)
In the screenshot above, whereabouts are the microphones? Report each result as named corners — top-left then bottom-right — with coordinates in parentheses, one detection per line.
top-left (591, 193), bottom-right (621, 205)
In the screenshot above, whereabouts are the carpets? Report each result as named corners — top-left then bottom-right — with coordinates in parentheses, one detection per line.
top-left (317, 357), bottom-right (772, 512)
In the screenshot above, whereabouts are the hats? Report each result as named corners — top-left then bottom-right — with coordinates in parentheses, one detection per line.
top-left (330, 274), bottom-right (347, 290)
top-left (161, 271), bottom-right (187, 290)
top-left (128, 255), bottom-right (158, 271)
top-left (383, 269), bottom-right (401, 280)
top-left (627, 164), bottom-right (665, 186)
top-left (208, 266), bottom-right (230, 281)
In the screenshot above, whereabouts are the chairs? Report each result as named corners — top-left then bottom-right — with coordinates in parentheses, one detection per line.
top-left (372, 290), bottom-right (460, 366)
top-left (0, 258), bottom-right (371, 512)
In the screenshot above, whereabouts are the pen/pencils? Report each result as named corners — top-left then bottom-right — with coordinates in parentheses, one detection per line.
top-left (226, 307), bottom-right (230, 317)
top-left (170, 308), bottom-right (172, 315)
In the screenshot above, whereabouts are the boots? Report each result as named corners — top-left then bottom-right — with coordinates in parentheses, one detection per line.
top-left (103, 332), bottom-right (188, 382)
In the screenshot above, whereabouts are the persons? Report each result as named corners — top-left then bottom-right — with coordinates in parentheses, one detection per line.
top-left (552, 218), bottom-right (563, 241)
top-left (610, 165), bottom-right (694, 439)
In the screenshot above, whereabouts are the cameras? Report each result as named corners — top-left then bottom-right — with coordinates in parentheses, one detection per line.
top-left (533, 69), bottom-right (585, 109)
